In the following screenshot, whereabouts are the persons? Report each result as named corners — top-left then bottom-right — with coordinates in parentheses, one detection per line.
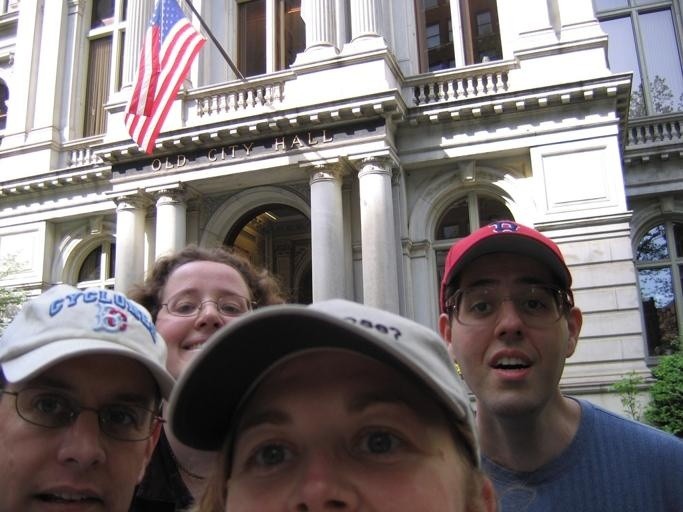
top-left (162, 296), bottom-right (496, 511)
top-left (0, 285), bottom-right (177, 512)
top-left (123, 241), bottom-right (285, 512)
top-left (437, 219), bottom-right (683, 511)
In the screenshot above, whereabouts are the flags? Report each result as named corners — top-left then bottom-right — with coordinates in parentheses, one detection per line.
top-left (122, 0), bottom-right (206, 157)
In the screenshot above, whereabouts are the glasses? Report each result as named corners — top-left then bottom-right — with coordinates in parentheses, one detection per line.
top-left (443, 283), bottom-right (570, 327)
top-left (0, 389), bottom-right (165, 442)
top-left (153, 294), bottom-right (257, 321)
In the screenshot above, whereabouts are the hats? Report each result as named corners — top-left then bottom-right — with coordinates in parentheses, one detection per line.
top-left (160, 294), bottom-right (480, 480)
top-left (439, 220), bottom-right (571, 317)
top-left (0, 283), bottom-right (177, 403)
